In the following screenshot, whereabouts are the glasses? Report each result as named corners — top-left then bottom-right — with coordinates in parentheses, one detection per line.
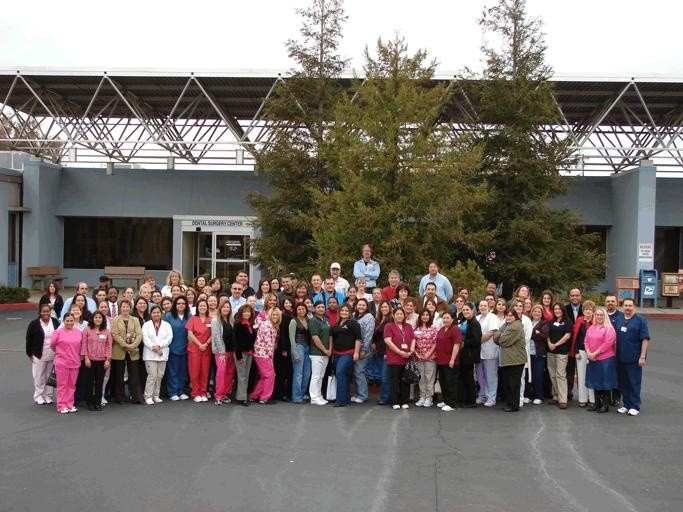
top-left (233, 288), bottom-right (242, 290)
top-left (177, 303), bottom-right (185, 305)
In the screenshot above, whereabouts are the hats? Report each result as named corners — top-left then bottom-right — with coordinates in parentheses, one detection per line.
top-left (331, 263), bottom-right (340, 269)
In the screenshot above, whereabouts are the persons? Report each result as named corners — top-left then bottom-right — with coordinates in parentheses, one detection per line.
top-left (419, 260), bottom-right (453, 306)
top-left (353, 244), bottom-right (381, 295)
top-left (321, 262), bottom-right (351, 299)
top-left (22, 270), bottom-right (652, 414)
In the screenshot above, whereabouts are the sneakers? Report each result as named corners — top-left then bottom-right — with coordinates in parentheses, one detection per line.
top-left (215, 394), bottom-right (275, 406)
top-left (100, 394), bottom-right (164, 406)
top-left (522, 397), bottom-right (567, 409)
top-left (37, 392), bottom-right (102, 413)
top-left (437, 402), bottom-right (456, 411)
top-left (169, 394), bottom-right (210, 403)
top-left (474, 396), bottom-right (497, 407)
top-left (276, 393), bottom-right (364, 406)
top-left (378, 397), bottom-right (435, 409)
top-left (579, 398), bottom-right (640, 416)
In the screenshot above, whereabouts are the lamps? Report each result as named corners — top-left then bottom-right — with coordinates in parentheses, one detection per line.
top-left (67, 141), bottom-right (262, 178)
top-left (575, 149), bottom-right (584, 169)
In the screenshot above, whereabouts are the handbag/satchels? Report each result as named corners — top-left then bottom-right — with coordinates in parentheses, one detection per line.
top-left (45, 372), bottom-right (57, 388)
top-left (400, 363), bottom-right (420, 384)
top-left (326, 375), bottom-right (337, 401)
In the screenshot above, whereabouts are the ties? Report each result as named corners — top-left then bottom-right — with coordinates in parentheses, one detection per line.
top-left (112, 305), bottom-right (116, 317)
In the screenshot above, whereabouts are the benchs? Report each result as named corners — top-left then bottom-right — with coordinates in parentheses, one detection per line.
top-left (26, 266), bottom-right (68, 292)
top-left (104, 265), bottom-right (146, 291)
